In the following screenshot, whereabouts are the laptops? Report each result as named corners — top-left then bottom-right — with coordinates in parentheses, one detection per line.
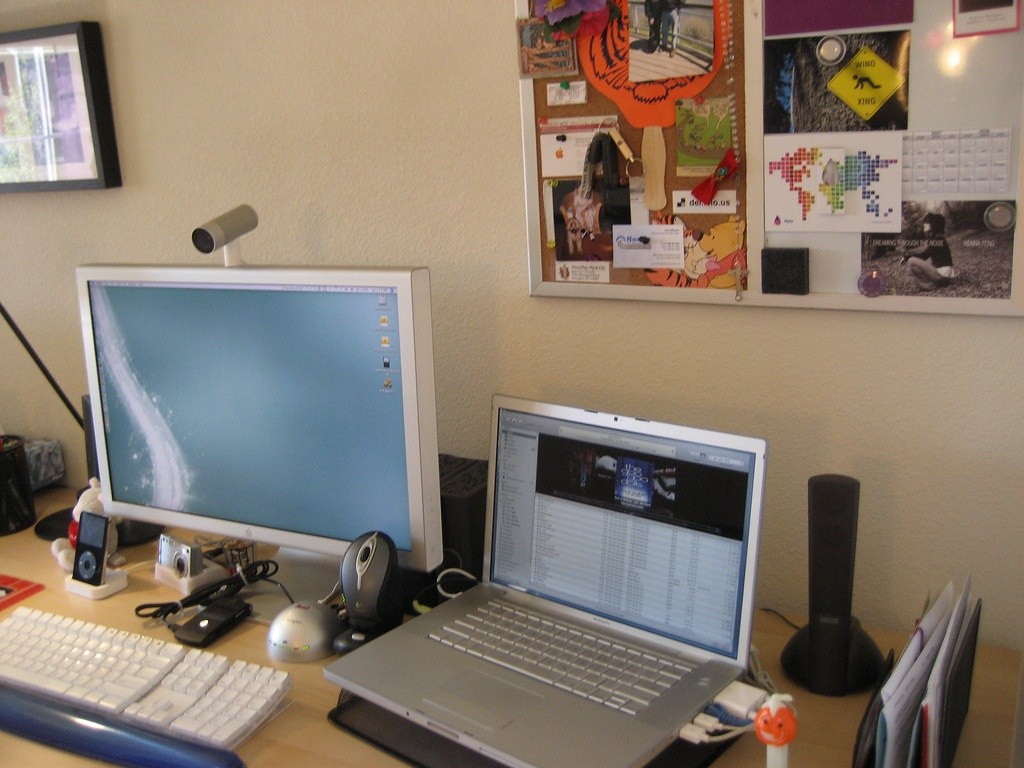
top-left (322, 394), bottom-right (767, 768)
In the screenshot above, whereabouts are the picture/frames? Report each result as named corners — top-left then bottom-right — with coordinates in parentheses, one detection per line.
top-left (0, 20), bottom-right (122, 194)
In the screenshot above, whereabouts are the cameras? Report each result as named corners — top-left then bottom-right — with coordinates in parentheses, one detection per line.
top-left (158, 532), bottom-right (203, 579)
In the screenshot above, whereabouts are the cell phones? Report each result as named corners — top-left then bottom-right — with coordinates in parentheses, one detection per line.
top-left (174, 595), bottom-right (253, 647)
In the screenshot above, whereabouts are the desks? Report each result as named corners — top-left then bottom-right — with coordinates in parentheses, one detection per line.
top-left (0, 486), bottom-right (1024, 768)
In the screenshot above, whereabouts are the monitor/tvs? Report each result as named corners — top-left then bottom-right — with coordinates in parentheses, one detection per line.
top-left (74, 265), bottom-right (443, 625)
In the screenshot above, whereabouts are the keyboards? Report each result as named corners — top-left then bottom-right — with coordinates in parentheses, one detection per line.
top-left (0, 606), bottom-right (291, 751)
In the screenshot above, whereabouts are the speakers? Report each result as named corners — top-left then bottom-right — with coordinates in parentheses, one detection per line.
top-left (780, 473), bottom-right (884, 697)
top-left (81, 393), bottom-right (166, 547)
top-left (408, 452), bottom-right (489, 607)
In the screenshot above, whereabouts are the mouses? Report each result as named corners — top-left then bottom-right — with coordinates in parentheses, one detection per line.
top-left (333, 530), bottom-right (401, 656)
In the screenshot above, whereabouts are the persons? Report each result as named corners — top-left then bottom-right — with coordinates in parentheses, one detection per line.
top-left (902, 213), bottom-right (956, 294)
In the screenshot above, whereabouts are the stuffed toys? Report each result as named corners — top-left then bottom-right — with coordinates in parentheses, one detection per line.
top-left (52, 477), bottom-right (120, 569)
